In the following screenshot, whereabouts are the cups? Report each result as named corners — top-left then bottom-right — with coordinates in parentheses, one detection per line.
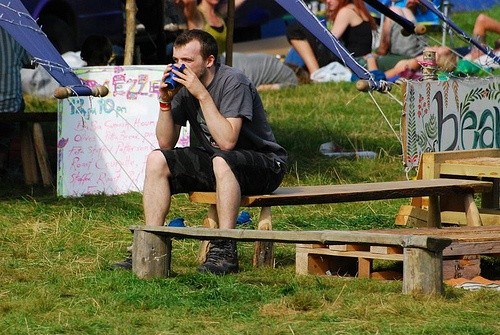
top-left (163, 64), bottom-right (185, 91)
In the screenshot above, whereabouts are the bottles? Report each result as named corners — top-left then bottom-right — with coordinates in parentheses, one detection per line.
top-left (423, 50), bottom-right (438, 81)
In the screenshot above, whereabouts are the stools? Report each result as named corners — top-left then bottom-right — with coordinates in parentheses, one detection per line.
top-left (0, 112), bottom-right (57, 189)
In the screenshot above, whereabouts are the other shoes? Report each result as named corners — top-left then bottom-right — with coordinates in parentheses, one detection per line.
top-left (108, 239), bottom-right (172, 277)
top-left (195, 238), bottom-right (239, 276)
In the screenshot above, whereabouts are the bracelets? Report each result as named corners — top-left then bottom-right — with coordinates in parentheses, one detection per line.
top-left (159, 96), bottom-right (172, 112)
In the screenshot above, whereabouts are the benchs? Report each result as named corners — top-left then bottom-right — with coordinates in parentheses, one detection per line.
top-left (126, 179), bottom-right (500, 300)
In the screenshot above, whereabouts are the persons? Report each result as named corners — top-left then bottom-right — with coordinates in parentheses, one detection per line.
top-left (0, 29), bottom-right (34, 196)
top-left (36, 0), bottom-right (81, 54)
top-left (325, 0), bottom-right (378, 58)
top-left (175, 0), bottom-right (296, 83)
top-left (376, 7), bottom-right (500, 71)
top-left (286, 25), bottom-right (456, 82)
top-left (82, 34), bottom-right (113, 66)
top-left (111, 29), bottom-right (287, 275)
